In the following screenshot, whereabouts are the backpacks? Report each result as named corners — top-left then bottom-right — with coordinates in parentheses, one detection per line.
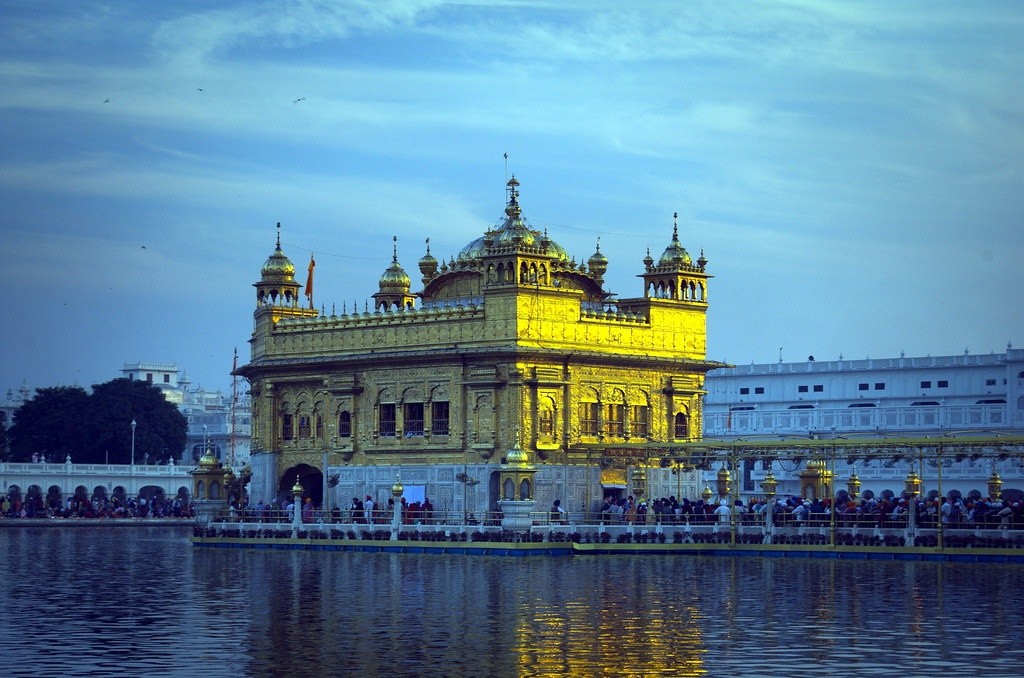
top-left (946, 501), bottom-right (959, 522)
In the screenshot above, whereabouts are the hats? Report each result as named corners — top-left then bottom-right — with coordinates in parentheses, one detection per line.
top-left (847, 501), bottom-right (855, 509)
top-left (967, 503), bottom-right (975, 508)
top-left (974, 497), bottom-right (1019, 509)
top-left (720, 500), bottom-right (727, 505)
top-left (803, 502), bottom-right (810, 506)
top-left (777, 497), bottom-right (787, 507)
top-left (898, 498), bottom-right (908, 504)
top-left (822, 498), bottom-right (828, 506)
top-left (752, 497), bottom-right (758, 504)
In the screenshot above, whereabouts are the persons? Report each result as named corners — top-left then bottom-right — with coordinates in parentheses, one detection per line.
top-left (229, 498), bottom-right (295, 523)
top-left (301, 495), bottom-right (394, 524)
top-left (834, 497), bottom-right (909, 528)
top-left (916, 496), bottom-right (1024, 530)
top-left (551, 500), bottom-right (564, 525)
top-left (734, 497), bottom-right (832, 527)
top-left (493, 510), bottom-right (504, 526)
top-left (601, 494), bottom-right (732, 525)
top-left (0, 492), bottom-right (195, 519)
top-left (467, 513), bottom-right (480, 526)
top-left (400, 497), bottom-right (433, 525)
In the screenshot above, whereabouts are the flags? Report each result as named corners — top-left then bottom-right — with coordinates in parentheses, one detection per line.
top-left (305, 261), bottom-right (313, 296)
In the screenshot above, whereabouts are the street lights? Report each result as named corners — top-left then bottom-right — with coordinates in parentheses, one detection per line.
top-left (201, 423), bottom-right (208, 456)
top-left (131, 418), bottom-right (137, 500)
